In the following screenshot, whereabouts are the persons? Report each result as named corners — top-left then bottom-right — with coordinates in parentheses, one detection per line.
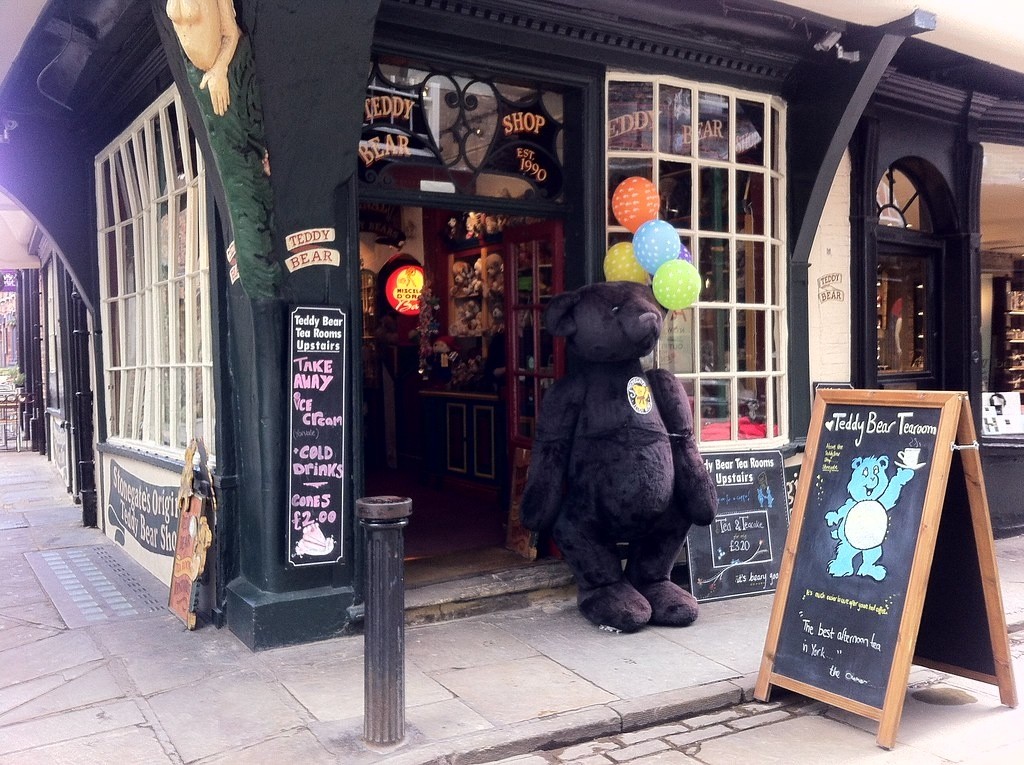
top-left (360, 343), bottom-right (385, 476)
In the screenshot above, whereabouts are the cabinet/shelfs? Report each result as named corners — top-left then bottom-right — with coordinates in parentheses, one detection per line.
top-left (449, 213), bottom-right (567, 387)
top-left (988, 276), bottom-right (1024, 392)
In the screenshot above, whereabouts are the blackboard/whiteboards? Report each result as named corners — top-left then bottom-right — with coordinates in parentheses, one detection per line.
top-left (287, 306), bottom-right (347, 568)
top-left (687, 450), bottom-right (789, 603)
top-left (763, 389), bottom-right (1011, 723)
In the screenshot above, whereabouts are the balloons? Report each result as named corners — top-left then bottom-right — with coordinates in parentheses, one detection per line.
top-left (605, 177), bottom-right (700, 310)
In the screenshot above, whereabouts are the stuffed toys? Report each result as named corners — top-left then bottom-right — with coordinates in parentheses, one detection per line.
top-left (519, 282), bottom-right (719, 632)
top-left (425, 211), bottom-right (548, 394)
